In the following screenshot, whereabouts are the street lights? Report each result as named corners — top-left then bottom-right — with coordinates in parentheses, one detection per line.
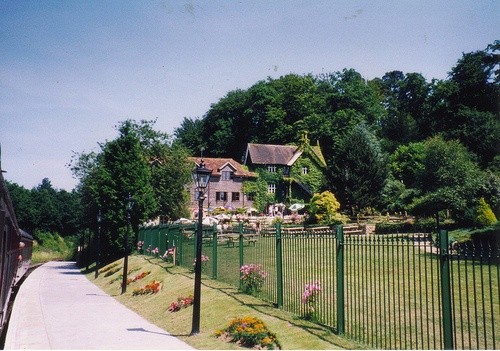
top-left (190, 159), bottom-right (215, 336)
top-left (95, 209), bottom-right (103, 279)
top-left (123, 193), bottom-right (136, 294)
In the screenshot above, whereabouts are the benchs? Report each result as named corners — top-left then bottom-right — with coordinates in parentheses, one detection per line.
top-left (182, 222), bottom-right (364, 248)
